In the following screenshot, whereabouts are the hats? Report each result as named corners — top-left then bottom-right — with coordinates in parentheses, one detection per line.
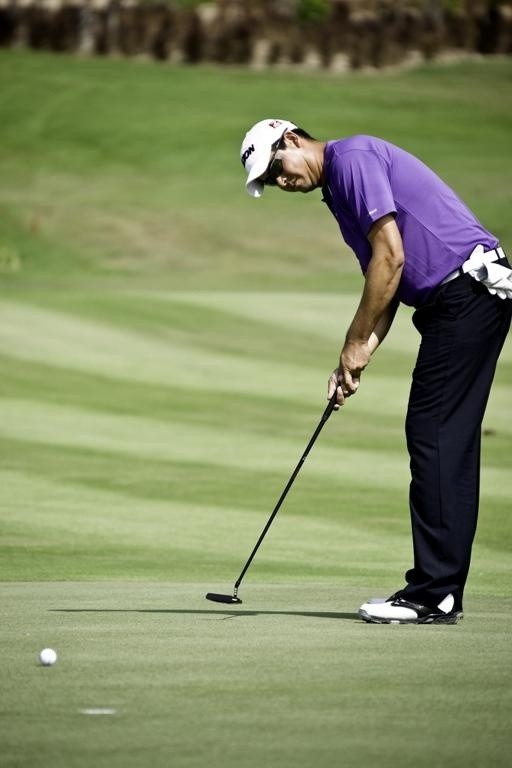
top-left (240, 118), bottom-right (299, 198)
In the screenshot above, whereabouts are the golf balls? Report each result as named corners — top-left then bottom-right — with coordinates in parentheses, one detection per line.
top-left (40, 648), bottom-right (57, 665)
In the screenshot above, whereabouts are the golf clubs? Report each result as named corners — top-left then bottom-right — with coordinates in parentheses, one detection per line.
top-left (207, 391), bottom-right (338, 603)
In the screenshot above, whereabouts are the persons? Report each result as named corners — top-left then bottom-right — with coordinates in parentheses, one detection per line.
top-left (239, 117), bottom-right (512, 624)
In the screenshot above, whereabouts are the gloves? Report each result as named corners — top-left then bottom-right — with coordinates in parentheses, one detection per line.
top-left (468, 244), bottom-right (512, 300)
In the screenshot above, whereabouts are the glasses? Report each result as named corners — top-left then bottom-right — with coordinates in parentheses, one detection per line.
top-left (261, 128), bottom-right (287, 185)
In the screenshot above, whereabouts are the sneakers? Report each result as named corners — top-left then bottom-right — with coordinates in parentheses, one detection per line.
top-left (358, 590), bottom-right (463, 624)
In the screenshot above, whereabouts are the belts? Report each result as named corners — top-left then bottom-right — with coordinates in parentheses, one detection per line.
top-left (441, 246), bottom-right (505, 285)
